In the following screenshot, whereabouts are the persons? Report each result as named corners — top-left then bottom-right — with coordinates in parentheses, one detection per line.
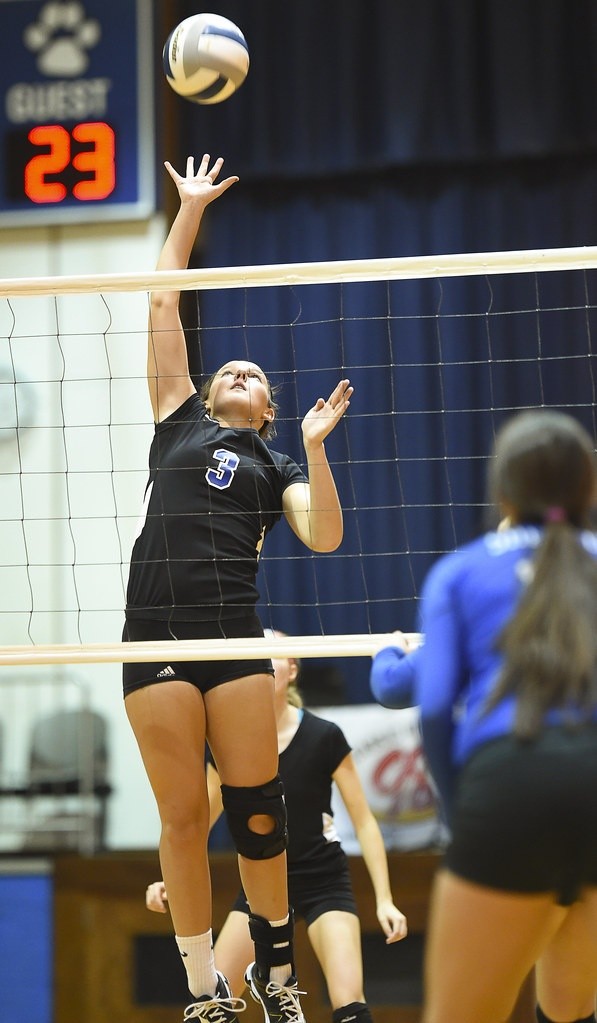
top-left (370, 411), bottom-right (597, 1023)
top-left (145, 628), bottom-right (409, 1023)
top-left (121, 153), bottom-right (355, 1023)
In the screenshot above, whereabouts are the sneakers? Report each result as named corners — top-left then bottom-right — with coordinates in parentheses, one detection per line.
top-left (244, 961), bottom-right (308, 1023)
top-left (183, 970), bottom-right (248, 1023)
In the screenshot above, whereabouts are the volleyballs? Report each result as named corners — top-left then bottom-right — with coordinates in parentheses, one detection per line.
top-left (162, 12), bottom-right (253, 106)
